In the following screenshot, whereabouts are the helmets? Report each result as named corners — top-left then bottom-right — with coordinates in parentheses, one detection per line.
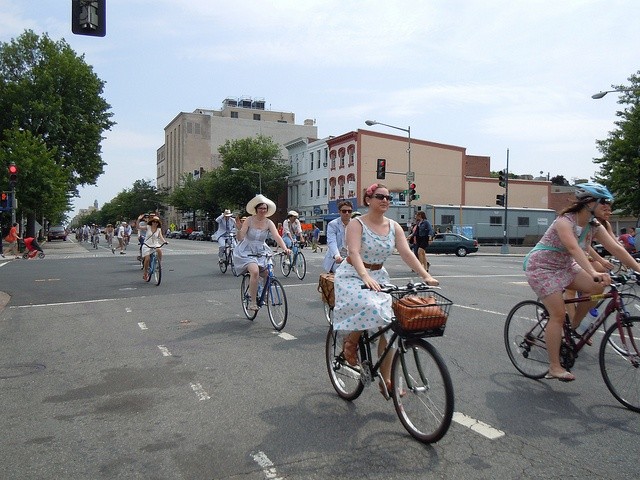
top-left (575, 182), bottom-right (611, 200)
top-left (144, 213), bottom-right (149, 216)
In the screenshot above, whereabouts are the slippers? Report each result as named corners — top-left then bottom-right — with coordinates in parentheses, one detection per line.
top-left (568, 325), bottom-right (592, 347)
top-left (543, 371), bottom-right (575, 380)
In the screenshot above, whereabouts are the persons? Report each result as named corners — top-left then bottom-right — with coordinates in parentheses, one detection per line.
top-left (149, 213), bottom-right (155, 220)
top-left (136, 216), bottom-right (168, 282)
top-left (282, 210), bottom-right (305, 266)
top-left (561, 198), bottom-right (625, 321)
top-left (616, 227), bottom-right (636, 256)
top-left (312, 223), bottom-right (323, 254)
top-left (334, 183), bottom-right (440, 398)
top-left (349, 208), bottom-right (361, 220)
top-left (408, 209), bottom-right (433, 274)
top-left (273, 222), bottom-right (283, 252)
top-left (323, 202), bottom-right (357, 274)
top-left (445, 226), bottom-right (453, 235)
top-left (112, 221), bottom-right (126, 256)
top-left (628, 227), bottom-right (636, 237)
top-left (435, 227), bottom-right (441, 235)
top-left (524, 183), bottom-right (640, 380)
top-left (238, 213), bottom-right (247, 223)
top-left (165, 227), bottom-right (170, 238)
top-left (211, 209), bottom-right (237, 262)
top-left (1, 223), bottom-right (23, 259)
top-left (124, 223), bottom-right (132, 239)
top-left (231, 194), bottom-right (292, 310)
top-left (138, 213), bottom-right (149, 247)
top-left (75, 223), bottom-right (114, 247)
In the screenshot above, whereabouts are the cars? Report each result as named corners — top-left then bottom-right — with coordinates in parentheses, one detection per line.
top-left (189, 231), bottom-right (200, 240)
top-left (426, 233), bottom-right (478, 256)
top-left (196, 231), bottom-right (213, 240)
top-left (47, 225), bottom-right (67, 242)
top-left (266, 231), bottom-right (277, 247)
top-left (168, 231), bottom-right (179, 239)
top-left (176, 230), bottom-right (190, 239)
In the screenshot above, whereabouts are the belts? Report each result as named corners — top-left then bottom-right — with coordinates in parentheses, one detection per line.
top-left (347, 255), bottom-right (384, 270)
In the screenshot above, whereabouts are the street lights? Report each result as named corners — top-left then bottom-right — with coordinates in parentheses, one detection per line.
top-left (143, 199), bottom-right (160, 213)
top-left (365, 120), bottom-right (410, 172)
top-left (592, 89), bottom-right (640, 102)
top-left (231, 168), bottom-right (262, 194)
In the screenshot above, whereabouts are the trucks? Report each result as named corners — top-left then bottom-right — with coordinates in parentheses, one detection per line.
top-left (305, 214), bottom-right (340, 244)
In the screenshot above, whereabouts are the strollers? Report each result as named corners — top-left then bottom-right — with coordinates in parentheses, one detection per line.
top-left (23, 237), bottom-right (45, 258)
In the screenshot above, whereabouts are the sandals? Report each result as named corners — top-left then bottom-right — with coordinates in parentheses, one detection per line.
top-left (343, 333), bottom-right (358, 366)
top-left (379, 381), bottom-right (405, 397)
top-left (251, 305), bottom-right (258, 311)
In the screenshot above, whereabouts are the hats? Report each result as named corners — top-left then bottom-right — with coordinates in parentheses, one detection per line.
top-left (149, 217), bottom-right (162, 228)
top-left (246, 194), bottom-right (276, 217)
top-left (122, 222), bottom-right (126, 224)
top-left (288, 211), bottom-right (298, 216)
top-left (222, 210), bottom-right (233, 216)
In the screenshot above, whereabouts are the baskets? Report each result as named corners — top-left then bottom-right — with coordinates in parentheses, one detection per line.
top-left (390, 290), bottom-right (452, 337)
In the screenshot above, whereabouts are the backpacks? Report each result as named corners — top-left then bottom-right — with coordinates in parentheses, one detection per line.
top-left (393, 296), bottom-right (446, 331)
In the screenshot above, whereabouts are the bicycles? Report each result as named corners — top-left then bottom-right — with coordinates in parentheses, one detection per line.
top-left (326, 282), bottom-right (454, 443)
top-left (281, 240), bottom-right (308, 280)
top-left (322, 257), bottom-right (347, 326)
top-left (106, 235), bottom-right (114, 248)
top-left (536, 259), bottom-right (640, 356)
top-left (123, 235), bottom-right (131, 250)
top-left (503, 272), bottom-right (640, 412)
top-left (609, 253), bottom-right (640, 280)
top-left (241, 251), bottom-right (287, 330)
top-left (219, 235), bottom-right (236, 276)
top-left (93, 232), bottom-right (100, 249)
top-left (140, 242), bottom-right (168, 286)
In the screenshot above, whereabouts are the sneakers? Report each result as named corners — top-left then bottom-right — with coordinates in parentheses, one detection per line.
top-left (284, 259), bottom-right (290, 265)
top-left (143, 274), bottom-right (149, 281)
top-left (120, 251), bottom-right (126, 254)
top-left (112, 249), bottom-right (115, 254)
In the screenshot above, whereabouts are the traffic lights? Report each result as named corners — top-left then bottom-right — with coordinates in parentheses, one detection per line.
top-left (10, 165), bottom-right (18, 189)
top-left (377, 159), bottom-right (386, 180)
top-left (496, 194), bottom-right (505, 205)
top-left (0, 191), bottom-right (10, 211)
top-left (409, 183), bottom-right (416, 200)
top-left (499, 171), bottom-right (506, 187)
top-left (72, 0), bottom-right (106, 37)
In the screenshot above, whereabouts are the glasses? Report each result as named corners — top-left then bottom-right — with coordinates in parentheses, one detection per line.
top-left (152, 220), bottom-right (159, 223)
top-left (592, 197), bottom-right (612, 205)
top-left (257, 207), bottom-right (267, 210)
top-left (371, 194), bottom-right (394, 201)
top-left (340, 209), bottom-right (353, 213)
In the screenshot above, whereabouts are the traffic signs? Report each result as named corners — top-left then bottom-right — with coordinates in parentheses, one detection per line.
top-left (407, 172), bottom-right (414, 182)
